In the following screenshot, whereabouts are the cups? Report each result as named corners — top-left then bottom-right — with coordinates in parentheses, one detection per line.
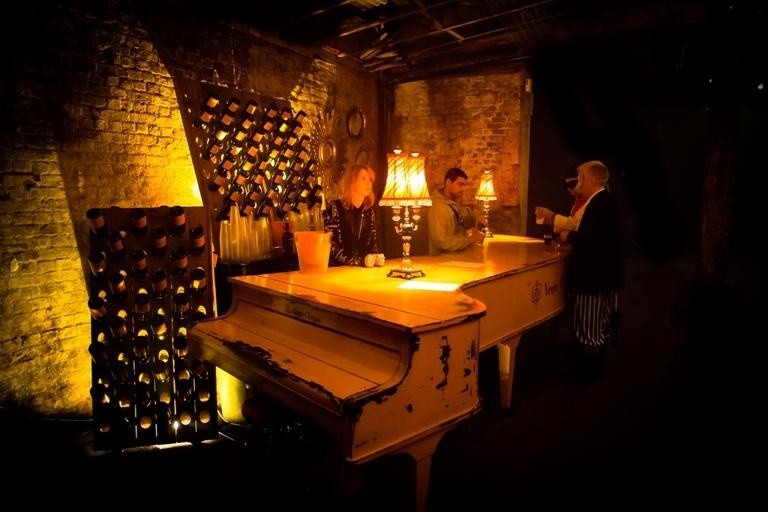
top-left (218, 207), bottom-right (271, 265)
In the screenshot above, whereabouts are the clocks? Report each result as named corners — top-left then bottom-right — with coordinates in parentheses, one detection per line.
top-left (311, 106), bottom-right (369, 189)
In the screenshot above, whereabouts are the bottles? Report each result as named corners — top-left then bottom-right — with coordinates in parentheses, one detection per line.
top-left (195, 91), bottom-right (323, 218)
top-left (282, 222), bottom-right (294, 253)
top-left (86, 206), bottom-right (212, 439)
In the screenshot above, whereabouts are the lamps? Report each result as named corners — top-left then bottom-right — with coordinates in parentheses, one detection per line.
top-left (378, 145), bottom-right (433, 280)
top-left (474, 168), bottom-right (497, 238)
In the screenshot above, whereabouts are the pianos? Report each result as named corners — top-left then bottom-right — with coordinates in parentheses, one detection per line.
top-left (188, 235), bottom-right (569, 512)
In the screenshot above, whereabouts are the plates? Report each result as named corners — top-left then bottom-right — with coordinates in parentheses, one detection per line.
top-left (347, 109), bottom-right (367, 140)
top-left (318, 139), bottom-right (337, 167)
top-left (355, 147), bottom-right (371, 169)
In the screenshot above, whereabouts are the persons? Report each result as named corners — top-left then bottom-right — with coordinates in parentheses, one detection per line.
top-left (318, 163), bottom-right (387, 268)
top-left (551, 158), bottom-right (623, 388)
top-left (530, 168), bottom-right (588, 248)
top-left (427, 167), bottom-right (488, 255)
